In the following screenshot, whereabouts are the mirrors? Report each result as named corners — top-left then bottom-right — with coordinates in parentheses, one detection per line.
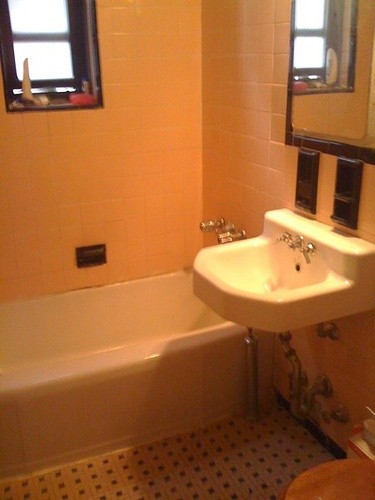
top-left (286, 0), bottom-right (375, 164)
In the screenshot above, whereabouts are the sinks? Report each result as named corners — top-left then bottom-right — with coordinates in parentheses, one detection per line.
top-left (194, 210), bottom-right (375, 332)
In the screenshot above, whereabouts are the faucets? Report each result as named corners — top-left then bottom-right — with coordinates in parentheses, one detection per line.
top-left (269, 232), bottom-right (317, 265)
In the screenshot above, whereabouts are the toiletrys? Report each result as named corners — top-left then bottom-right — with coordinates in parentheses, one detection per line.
top-left (325, 48), bottom-right (338, 88)
top-left (21, 57), bottom-right (34, 106)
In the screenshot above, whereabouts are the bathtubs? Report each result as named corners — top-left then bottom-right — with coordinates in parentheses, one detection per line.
top-left (0, 268), bottom-right (276, 475)
